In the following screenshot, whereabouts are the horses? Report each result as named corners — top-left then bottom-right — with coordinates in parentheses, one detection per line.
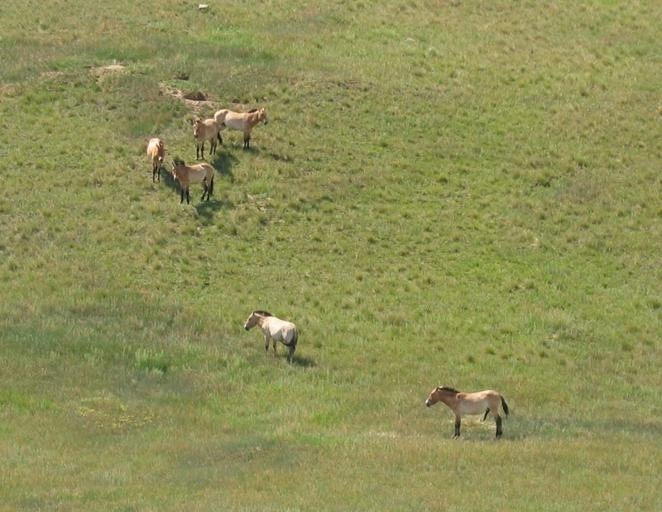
top-left (214, 107), bottom-right (268, 150)
top-left (243, 310), bottom-right (299, 364)
top-left (424, 384), bottom-right (509, 441)
top-left (170, 154), bottom-right (215, 206)
top-left (186, 113), bottom-right (221, 161)
top-left (146, 137), bottom-right (166, 183)
